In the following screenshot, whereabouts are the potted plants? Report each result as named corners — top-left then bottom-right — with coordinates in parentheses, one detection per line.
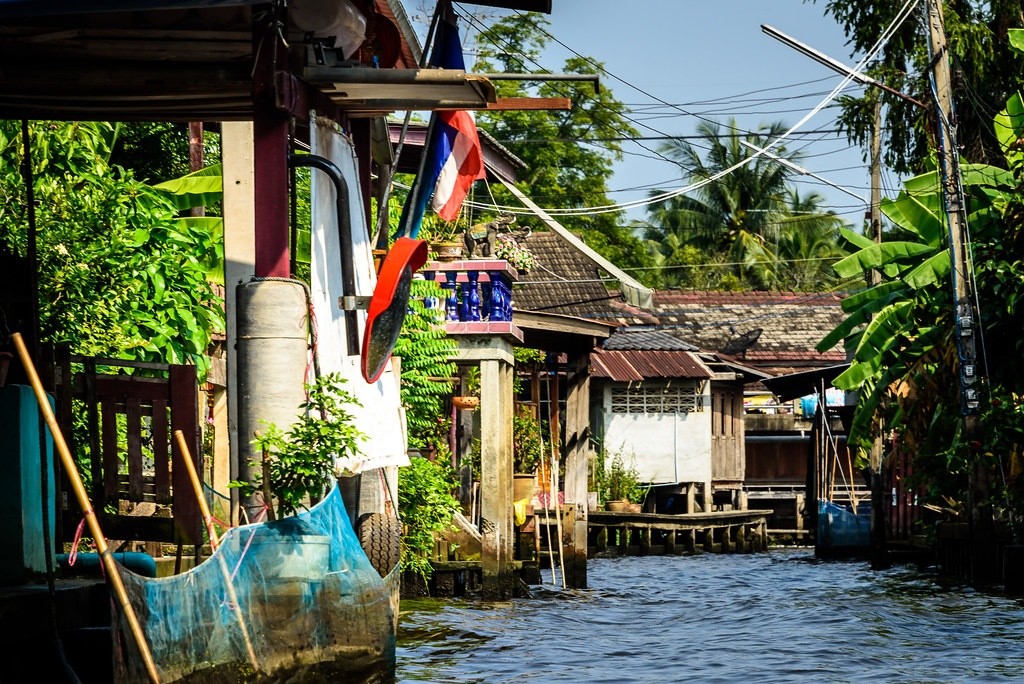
top-left (430, 230), bottom-right (463, 260)
top-left (513, 404), bottom-right (549, 512)
top-left (606, 443), bottom-right (652, 514)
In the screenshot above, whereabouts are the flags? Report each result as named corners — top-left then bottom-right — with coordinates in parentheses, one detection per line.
top-left (422, 0), bottom-right (488, 220)
